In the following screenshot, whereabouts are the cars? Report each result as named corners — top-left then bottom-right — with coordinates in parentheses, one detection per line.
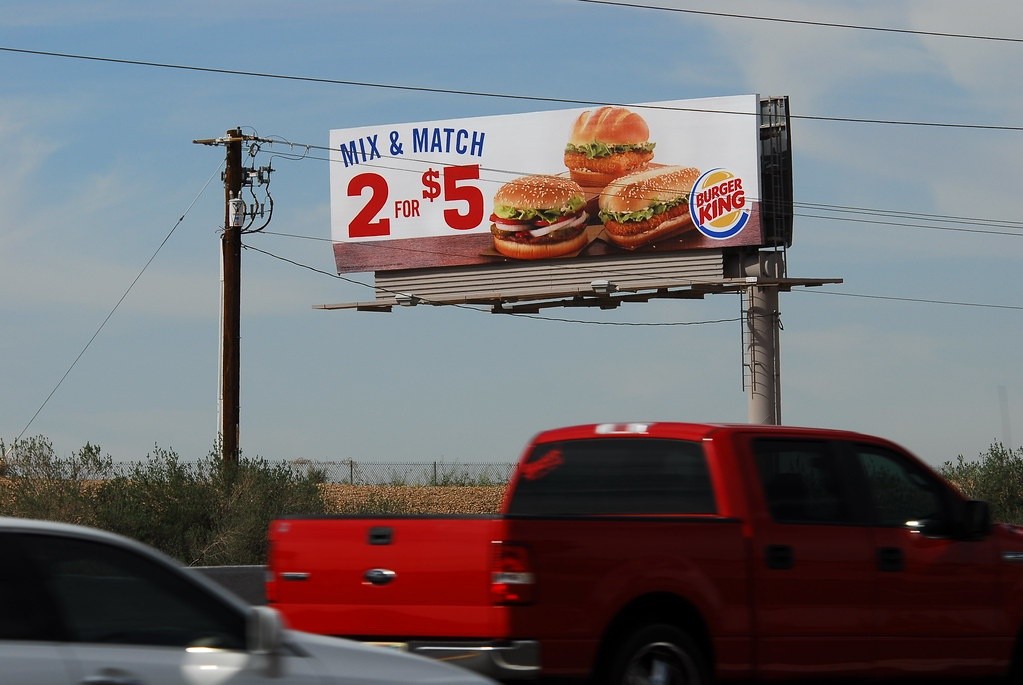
top-left (0, 515), bottom-right (498, 685)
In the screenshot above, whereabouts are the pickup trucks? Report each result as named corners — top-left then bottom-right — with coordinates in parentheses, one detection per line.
top-left (263, 418), bottom-right (1023, 684)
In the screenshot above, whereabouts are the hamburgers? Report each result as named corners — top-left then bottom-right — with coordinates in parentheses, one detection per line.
top-left (488, 107), bottom-right (702, 261)
